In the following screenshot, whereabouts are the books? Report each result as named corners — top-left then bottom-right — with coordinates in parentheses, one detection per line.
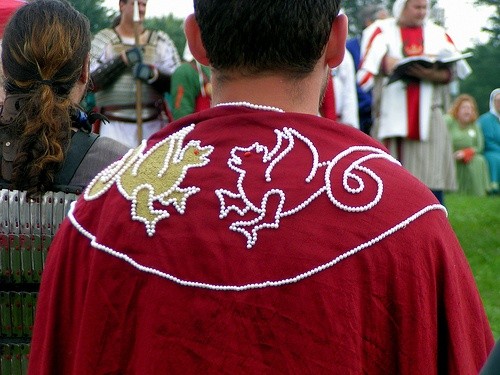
top-left (387, 52), bottom-right (473, 85)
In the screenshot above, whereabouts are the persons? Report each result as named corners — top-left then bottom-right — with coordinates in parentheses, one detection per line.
top-left (0, 0), bottom-right (30, 105)
top-left (318, 7), bottom-right (360, 129)
top-left (345, 6), bottom-right (393, 135)
top-left (0, 0), bottom-right (131, 203)
top-left (27, 0), bottom-right (496, 375)
top-left (86, 0), bottom-right (181, 148)
top-left (477, 88), bottom-right (500, 195)
top-left (355, 0), bottom-right (473, 206)
top-left (170, 40), bottom-right (212, 120)
top-left (444, 94), bottom-right (489, 196)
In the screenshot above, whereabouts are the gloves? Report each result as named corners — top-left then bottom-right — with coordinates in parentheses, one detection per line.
top-left (131, 61), bottom-right (154, 83)
top-left (126, 47), bottom-right (146, 67)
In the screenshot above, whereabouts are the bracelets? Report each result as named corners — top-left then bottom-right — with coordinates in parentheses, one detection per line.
top-left (463, 148), bottom-right (474, 162)
